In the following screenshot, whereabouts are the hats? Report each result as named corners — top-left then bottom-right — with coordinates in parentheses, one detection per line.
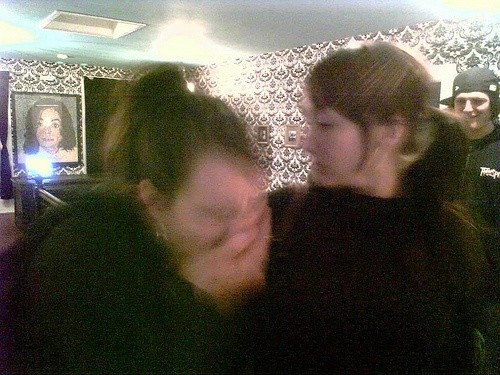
top-left (439, 68), bottom-right (500, 107)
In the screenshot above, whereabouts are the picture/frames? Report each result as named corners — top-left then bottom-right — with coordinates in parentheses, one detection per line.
top-left (257, 124), bottom-right (270, 146)
top-left (284, 124), bottom-right (300, 148)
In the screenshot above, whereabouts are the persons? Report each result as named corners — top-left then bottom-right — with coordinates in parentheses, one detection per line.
top-left (241, 43), bottom-right (491, 375)
top-left (18, 98), bottom-right (80, 163)
top-left (433, 67), bottom-right (500, 237)
top-left (0, 67), bottom-right (291, 375)
